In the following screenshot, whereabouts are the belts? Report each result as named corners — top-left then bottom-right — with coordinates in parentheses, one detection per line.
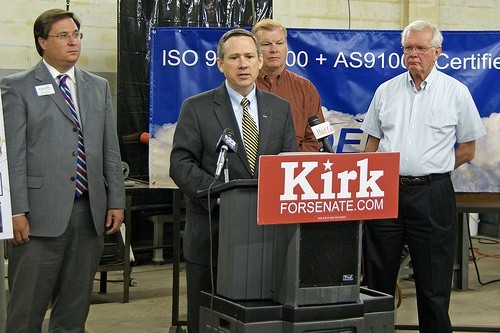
top-left (399, 172), bottom-right (449, 185)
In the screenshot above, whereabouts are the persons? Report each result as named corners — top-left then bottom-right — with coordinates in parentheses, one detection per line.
top-left (252, 19), bottom-right (326, 150)
top-left (0, 9), bottom-right (126, 333)
top-left (170, 28), bottom-right (298, 333)
top-left (360, 19), bottom-right (487, 333)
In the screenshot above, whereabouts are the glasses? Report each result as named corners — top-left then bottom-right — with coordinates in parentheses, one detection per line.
top-left (400, 46), bottom-right (435, 54)
top-left (47, 32), bottom-right (84, 40)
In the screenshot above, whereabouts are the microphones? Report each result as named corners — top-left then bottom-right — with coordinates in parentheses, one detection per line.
top-left (308, 115), bottom-right (336, 153)
top-left (213, 129), bottom-right (238, 180)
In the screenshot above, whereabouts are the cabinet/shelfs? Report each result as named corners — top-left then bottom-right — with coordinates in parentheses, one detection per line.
top-left (90, 188), bottom-right (134, 304)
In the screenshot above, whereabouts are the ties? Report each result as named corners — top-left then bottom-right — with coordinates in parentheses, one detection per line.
top-left (56, 74), bottom-right (88, 197)
top-left (240, 98), bottom-right (260, 177)
